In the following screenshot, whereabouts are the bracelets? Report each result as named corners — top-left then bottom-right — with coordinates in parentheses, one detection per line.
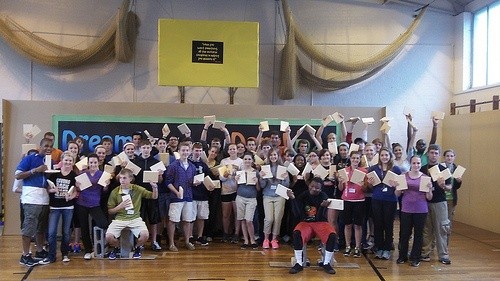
top-left (152, 187), bottom-right (158, 188)
top-left (31, 169), bottom-right (34, 175)
top-left (203, 128), bottom-right (208, 131)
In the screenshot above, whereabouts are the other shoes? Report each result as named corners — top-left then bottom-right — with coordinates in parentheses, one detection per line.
top-left (318, 245), bottom-right (322, 250)
top-left (231, 234), bottom-right (239, 243)
top-left (221, 236), bottom-right (228, 242)
top-left (251, 244), bottom-right (258, 248)
top-left (185, 242), bottom-right (195, 250)
top-left (240, 244), bottom-right (250, 249)
top-left (333, 245), bottom-right (339, 251)
top-left (169, 245), bottom-right (178, 252)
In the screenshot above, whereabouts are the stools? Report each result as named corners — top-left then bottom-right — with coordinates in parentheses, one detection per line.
top-left (94, 226), bottom-right (134, 259)
top-left (302, 236), bottom-right (334, 267)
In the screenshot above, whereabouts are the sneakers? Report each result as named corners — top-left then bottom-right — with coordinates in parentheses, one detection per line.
top-left (411, 260), bottom-right (420, 266)
top-left (322, 263), bottom-right (336, 274)
top-left (375, 250), bottom-right (383, 258)
top-left (263, 239), bottom-right (270, 248)
top-left (151, 240), bottom-right (162, 251)
top-left (420, 256), bottom-right (430, 261)
top-left (381, 250), bottom-right (391, 259)
top-left (353, 248), bottom-right (361, 258)
top-left (343, 246), bottom-right (351, 256)
top-left (189, 238), bottom-right (194, 245)
top-left (440, 258), bottom-right (451, 264)
top-left (397, 258), bottom-right (406, 263)
top-left (271, 239), bottom-right (278, 248)
top-left (289, 264), bottom-right (303, 273)
top-left (20, 240), bottom-right (144, 267)
top-left (196, 237), bottom-right (209, 245)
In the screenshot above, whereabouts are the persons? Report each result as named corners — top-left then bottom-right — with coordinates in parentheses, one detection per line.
top-left (12, 111), bottom-right (466, 267)
top-left (287, 177), bottom-right (338, 274)
